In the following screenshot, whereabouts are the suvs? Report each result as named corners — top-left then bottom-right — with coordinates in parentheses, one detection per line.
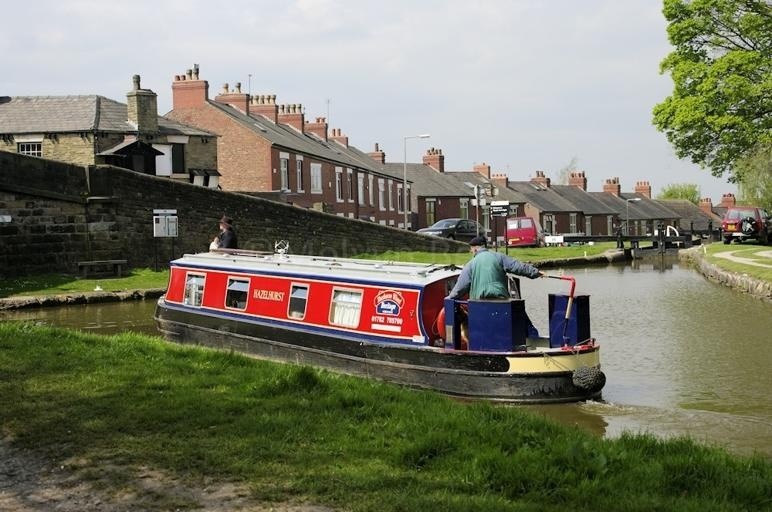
top-left (722, 207), bottom-right (770, 245)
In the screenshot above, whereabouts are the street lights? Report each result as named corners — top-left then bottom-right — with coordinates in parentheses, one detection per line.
top-left (476, 180), bottom-right (491, 242)
top-left (403, 134), bottom-right (430, 231)
top-left (626, 198), bottom-right (641, 236)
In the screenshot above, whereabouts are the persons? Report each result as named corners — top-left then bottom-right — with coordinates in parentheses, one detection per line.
top-left (444, 236), bottom-right (545, 301)
top-left (216, 215), bottom-right (239, 248)
top-left (209, 235), bottom-right (220, 250)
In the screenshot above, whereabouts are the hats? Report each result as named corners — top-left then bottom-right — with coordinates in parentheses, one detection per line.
top-left (216, 215), bottom-right (234, 227)
top-left (469, 237), bottom-right (487, 246)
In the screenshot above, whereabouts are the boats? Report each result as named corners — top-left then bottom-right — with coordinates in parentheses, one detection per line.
top-left (156, 250), bottom-right (606, 405)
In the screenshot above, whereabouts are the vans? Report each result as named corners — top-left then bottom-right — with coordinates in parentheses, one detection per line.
top-left (504, 216), bottom-right (544, 249)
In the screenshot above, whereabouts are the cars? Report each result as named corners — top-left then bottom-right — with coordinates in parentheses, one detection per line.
top-left (418, 218), bottom-right (487, 245)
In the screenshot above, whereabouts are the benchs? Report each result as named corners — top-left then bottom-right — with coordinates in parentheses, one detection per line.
top-left (76, 258), bottom-right (129, 278)
